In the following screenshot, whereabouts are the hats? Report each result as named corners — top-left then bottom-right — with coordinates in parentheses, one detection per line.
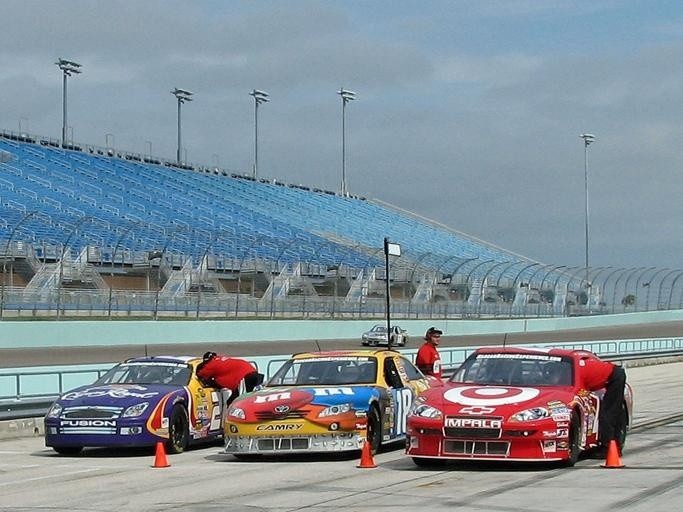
top-left (203, 352), bottom-right (216, 363)
top-left (427, 327), bottom-right (442, 337)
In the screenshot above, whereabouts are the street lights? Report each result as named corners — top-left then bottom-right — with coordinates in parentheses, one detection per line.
top-left (578, 132), bottom-right (596, 307)
top-left (334, 87), bottom-right (356, 198)
top-left (249, 88), bottom-right (270, 178)
top-left (169, 86), bottom-right (194, 166)
top-left (54, 57), bottom-right (82, 145)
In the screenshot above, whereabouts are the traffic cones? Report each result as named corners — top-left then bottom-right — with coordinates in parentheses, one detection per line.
top-left (152, 441), bottom-right (170, 468)
top-left (601, 440), bottom-right (626, 469)
top-left (355, 441), bottom-right (377, 470)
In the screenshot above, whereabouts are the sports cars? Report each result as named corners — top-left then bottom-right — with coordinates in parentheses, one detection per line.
top-left (45, 356), bottom-right (245, 452)
top-left (228, 350), bottom-right (438, 457)
top-left (406, 347), bottom-right (633, 466)
top-left (362, 324), bottom-right (409, 346)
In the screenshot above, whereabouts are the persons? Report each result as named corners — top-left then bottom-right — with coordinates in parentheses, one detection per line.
top-left (415, 326), bottom-right (444, 380)
top-left (196, 351), bottom-right (264, 408)
top-left (547, 346), bottom-right (627, 461)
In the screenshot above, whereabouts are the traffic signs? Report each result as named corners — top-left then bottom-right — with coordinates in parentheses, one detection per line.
top-left (381, 237), bottom-right (401, 350)
